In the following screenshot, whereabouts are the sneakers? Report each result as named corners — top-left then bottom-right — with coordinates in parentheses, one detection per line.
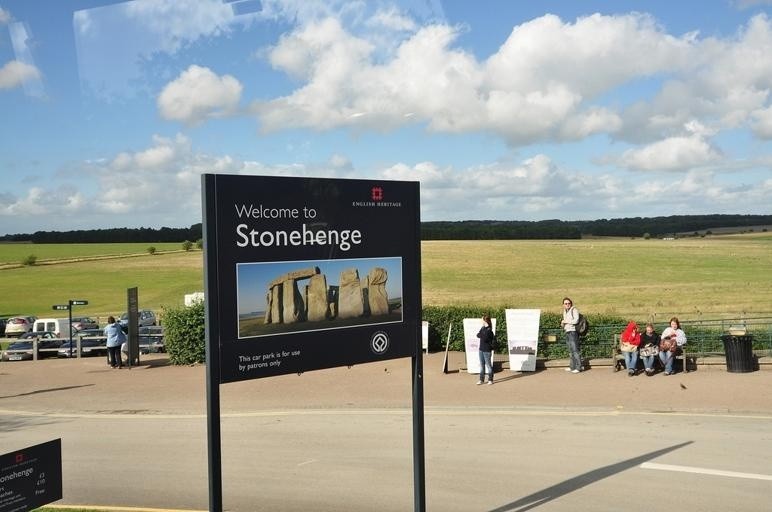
top-left (628, 368), bottom-right (676, 375)
top-left (566, 367), bottom-right (579, 373)
top-left (477, 380), bottom-right (493, 385)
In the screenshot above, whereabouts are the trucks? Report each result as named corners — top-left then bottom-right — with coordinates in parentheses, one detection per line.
top-left (34, 316), bottom-right (80, 338)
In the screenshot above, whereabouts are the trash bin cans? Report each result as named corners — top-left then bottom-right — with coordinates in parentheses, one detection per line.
top-left (722, 335), bottom-right (754, 372)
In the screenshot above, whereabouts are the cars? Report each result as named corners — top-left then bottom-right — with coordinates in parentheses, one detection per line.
top-left (72, 316), bottom-right (100, 332)
top-left (0, 318), bottom-right (8, 337)
top-left (2, 331), bottom-right (66, 360)
top-left (124, 325), bottom-right (167, 354)
top-left (5, 316), bottom-right (39, 338)
top-left (58, 328), bottom-right (109, 358)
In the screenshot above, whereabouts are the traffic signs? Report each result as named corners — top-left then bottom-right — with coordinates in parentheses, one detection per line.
top-left (51, 305), bottom-right (68, 310)
top-left (71, 301), bottom-right (87, 305)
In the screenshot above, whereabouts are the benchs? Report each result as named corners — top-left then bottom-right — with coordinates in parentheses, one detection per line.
top-left (612, 331), bottom-right (688, 376)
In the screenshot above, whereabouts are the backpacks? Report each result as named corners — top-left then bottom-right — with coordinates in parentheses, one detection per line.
top-left (571, 307), bottom-right (588, 335)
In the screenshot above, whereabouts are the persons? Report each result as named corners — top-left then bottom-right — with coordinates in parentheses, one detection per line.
top-left (103, 315), bottom-right (126, 370)
top-left (560, 297), bottom-right (586, 374)
top-left (638, 324), bottom-right (660, 376)
top-left (620, 321), bottom-right (640, 376)
top-left (658, 317), bottom-right (688, 375)
top-left (475, 315), bottom-right (495, 387)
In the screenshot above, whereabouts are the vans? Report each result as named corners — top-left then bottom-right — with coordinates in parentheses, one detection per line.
top-left (117, 309), bottom-right (156, 334)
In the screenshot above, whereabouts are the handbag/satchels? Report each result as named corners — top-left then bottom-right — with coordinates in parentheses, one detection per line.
top-left (621, 339), bottom-right (676, 357)
